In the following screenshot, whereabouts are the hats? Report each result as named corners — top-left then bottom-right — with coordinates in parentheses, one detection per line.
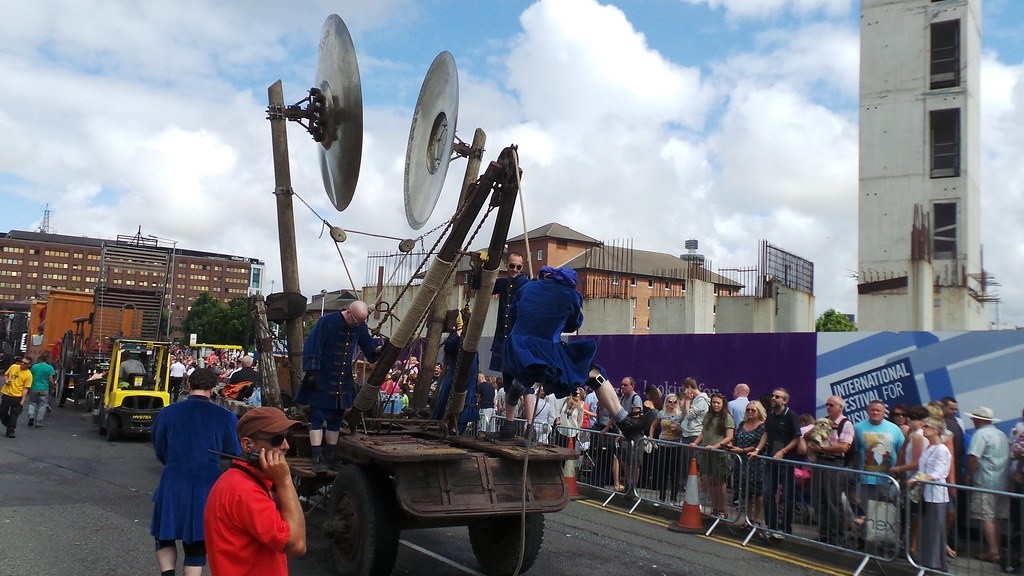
top-left (236, 406), bottom-right (303, 439)
top-left (964, 407), bottom-right (999, 422)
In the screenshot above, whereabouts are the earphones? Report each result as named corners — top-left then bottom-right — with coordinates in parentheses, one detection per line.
top-left (246, 442), bottom-right (251, 448)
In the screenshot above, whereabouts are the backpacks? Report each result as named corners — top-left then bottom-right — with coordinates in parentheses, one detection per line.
top-left (825, 414), bottom-right (856, 468)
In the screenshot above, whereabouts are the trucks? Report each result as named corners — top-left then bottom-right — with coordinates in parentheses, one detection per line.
top-left (211, 352), bottom-right (581, 576)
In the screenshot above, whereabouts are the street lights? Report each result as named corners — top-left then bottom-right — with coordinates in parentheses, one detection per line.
top-left (320, 289), bottom-right (327, 318)
top-left (269, 279), bottom-right (274, 294)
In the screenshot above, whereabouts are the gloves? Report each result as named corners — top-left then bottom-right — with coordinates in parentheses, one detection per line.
top-left (475, 250), bottom-right (489, 262)
top-left (307, 372), bottom-right (318, 391)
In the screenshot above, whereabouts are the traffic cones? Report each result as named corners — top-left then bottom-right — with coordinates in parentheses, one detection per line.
top-left (668, 458), bottom-right (707, 535)
top-left (564, 438), bottom-right (589, 500)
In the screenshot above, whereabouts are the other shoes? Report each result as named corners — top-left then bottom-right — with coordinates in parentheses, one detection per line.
top-left (580, 476), bottom-right (1024, 563)
top-left (484, 425), bottom-right (515, 443)
top-left (5, 427), bottom-right (16, 437)
top-left (522, 428), bottom-right (536, 447)
top-left (46, 405), bottom-right (52, 412)
top-left (619, 410), bottom-right (658, 441)
top-left (35, 420), bottom-right (44, 426)
top-left (28, 417), bottom-right (34, 426)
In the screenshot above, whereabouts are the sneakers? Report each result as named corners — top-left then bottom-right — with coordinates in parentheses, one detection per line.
top-left (323, 454), bottom-right (346, 470)
top-left (312, 453), bottom-right (325, 472)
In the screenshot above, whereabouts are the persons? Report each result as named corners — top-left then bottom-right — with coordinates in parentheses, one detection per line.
top-left (102, 350), bottom-right (146, 384)
top-left (204, 406), bottom-right (306, 576)
top-left (150, 368), bottom-right (242, 576)
top-left (170, 345), bottom-right (262, 387)
top-left (680, 378), bottom-right (854, 550)
top-left (381, 357), bottom-right (443, 409)
top-left (28, 351), bottom-right (56, 428)
top-left (1005, 409), bottom-right (1024, 575)
top-left (168, 358), bottom-right (187, 403)
top-left (963, 406), bottom-right (1009, 563)
top-left (470, 251), bottom-right (659, 449)
top-left (0, 356), bottom-right (33, 438)
top-left (494, 377), bottom-right (684, 507)
top-left (294, 300), bottom-right (390, 475)
top-left (476, 374), bottom-right (496, 436)
top-left (432, 308), bottom-right (480, 436)
top-left (854, 396), bottom-right (964, 559)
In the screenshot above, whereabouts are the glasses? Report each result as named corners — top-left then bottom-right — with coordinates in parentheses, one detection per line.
top-left (668, 400), bottom-right (678, 403)
top-left (507, 263), bottom-right (523, 270)
top-left (350, 312), bottom-right (363, 326)
top-left (826, 403), bottom-right (838, 407)
top-left (922, 425), bottom-right (939, 429)
top-left (970, 417), bottom-right (978, 421)
top-left (246, 431), bottom-right (294, 447)
top-left (746, 408), bottom-right (758, 412)
top-left (620, 383), bottom-right (631, 387)
top-left (892, 412), bottom-right (906, 417)
top-left (770, 394), bottom-right (784, 400)
top-left (21, 361), bottom-right (29, 365)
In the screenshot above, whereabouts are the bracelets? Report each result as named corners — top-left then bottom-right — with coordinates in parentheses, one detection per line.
top-left (782, 448), bottom-right (787, 453)
top-left (755, 448), bottom-right (760, 452)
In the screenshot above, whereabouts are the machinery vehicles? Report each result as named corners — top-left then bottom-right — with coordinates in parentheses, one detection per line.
top-left (0, 225), bottom-right (172, 442)
